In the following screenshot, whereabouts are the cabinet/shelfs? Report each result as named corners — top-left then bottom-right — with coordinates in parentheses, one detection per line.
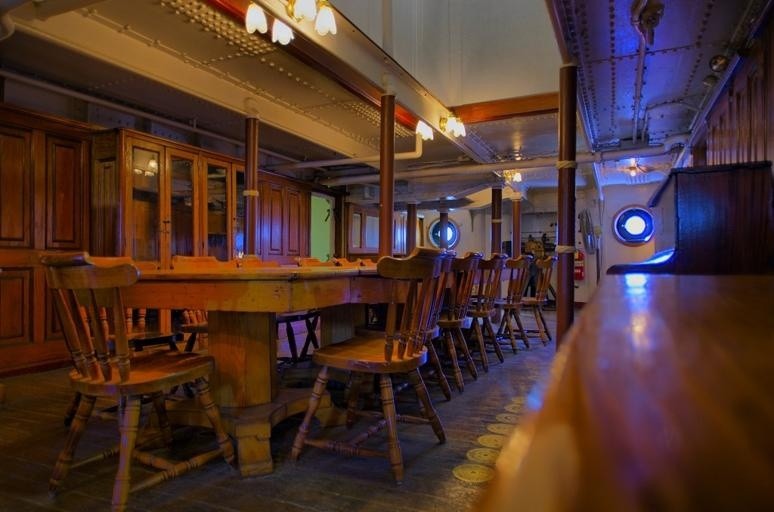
top-left (88, 126), bottom-right (245, 352)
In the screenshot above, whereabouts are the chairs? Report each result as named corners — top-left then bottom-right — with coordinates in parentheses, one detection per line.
top-left (83, 245), bottom-right (375, 401)
top-left (436, 248), bottom-right (556, 394)
top-left (37, 245), bottom-right (239, 512)
top-left (289, 244), bottom-right (448, 484)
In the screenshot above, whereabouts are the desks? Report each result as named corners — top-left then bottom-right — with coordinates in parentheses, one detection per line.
top-left (87, 262), bottom-right (519, 477)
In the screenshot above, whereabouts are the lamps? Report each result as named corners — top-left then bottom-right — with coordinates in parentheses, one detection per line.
top-left (708, 53), bottom-right (729, 73)
top-left (440, 112), bottom-right (467, 138)
top-left (286, 0), bottom-right (337, 36)
top-left (701, 74), bottom-right (724, 87)
top-left (244, 0), bottom-right (294, 46)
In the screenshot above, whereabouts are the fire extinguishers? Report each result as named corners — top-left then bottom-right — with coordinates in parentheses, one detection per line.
top-left (574, 248), bottom-right (584, 280)
